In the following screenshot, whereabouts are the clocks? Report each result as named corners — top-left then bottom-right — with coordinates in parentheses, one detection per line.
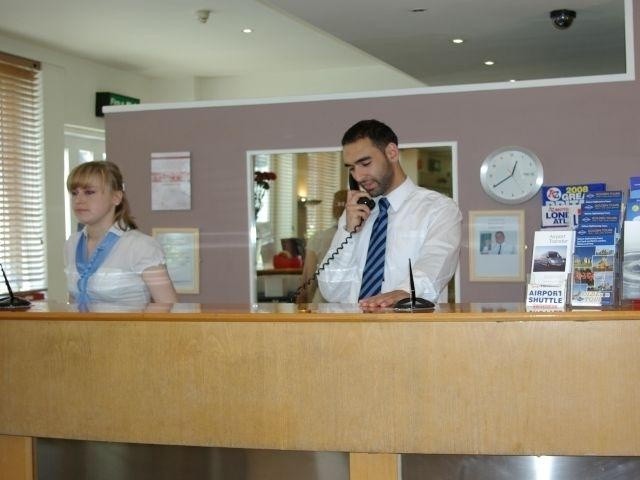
top-left (479, 146), bottom-right (544, 205)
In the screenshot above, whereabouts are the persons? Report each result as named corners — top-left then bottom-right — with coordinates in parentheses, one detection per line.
top-left (66, 161), bottom-right (181, 303)
top-left (298, 189), bottom-right (349, 304)
top-left (488, 231), bottom-right (516, 254)
top-left (313, 118), bottom-right (462, 307)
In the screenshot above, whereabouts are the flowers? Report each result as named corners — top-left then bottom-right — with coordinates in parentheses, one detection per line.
top-left (254, 171), bottom-right (276, 218)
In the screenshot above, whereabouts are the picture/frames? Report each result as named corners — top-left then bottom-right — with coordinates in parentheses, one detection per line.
top-left (468, 210), bottom-right (526, 282)
top-left (152, 227), bottom-right (201, 295)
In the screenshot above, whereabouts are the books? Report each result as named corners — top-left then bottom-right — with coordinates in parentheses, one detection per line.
top-left (523, 175), bottom-right (640, 307)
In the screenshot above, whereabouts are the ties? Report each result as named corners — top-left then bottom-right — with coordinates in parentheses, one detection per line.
top-left (358, 196), bottom-right (390, 302)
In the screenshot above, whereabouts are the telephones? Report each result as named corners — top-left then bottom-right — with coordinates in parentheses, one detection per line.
top-left (349, 175), bottom-right (375, 210)
